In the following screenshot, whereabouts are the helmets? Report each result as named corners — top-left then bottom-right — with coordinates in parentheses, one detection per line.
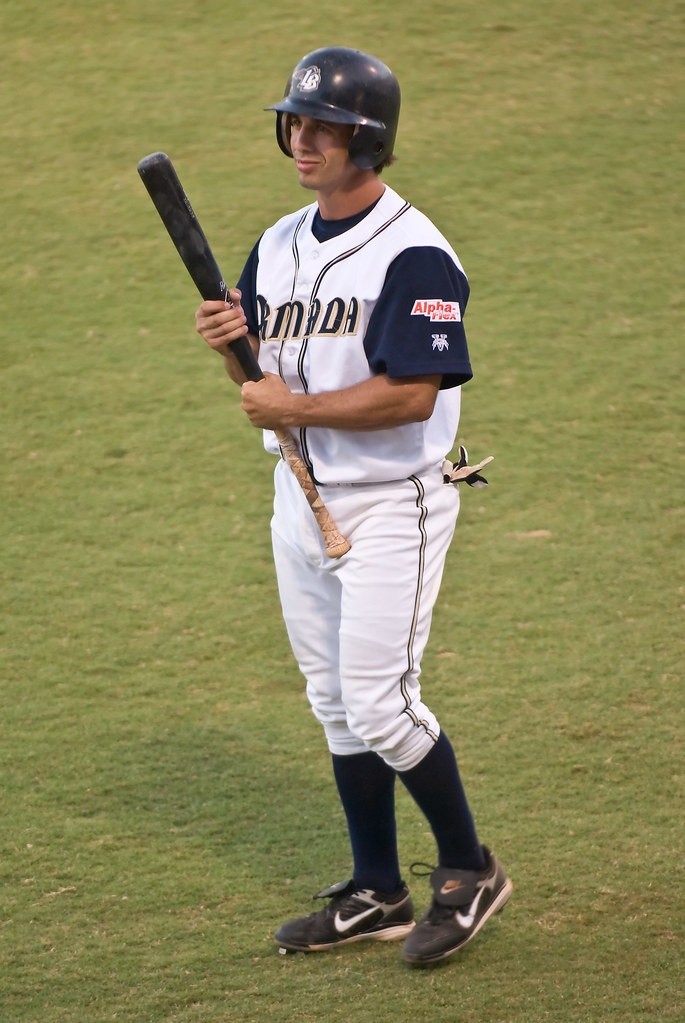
top-left (263, 45), bottom-right (401, 171)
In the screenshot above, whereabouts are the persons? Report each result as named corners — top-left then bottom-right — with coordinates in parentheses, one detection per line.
top-left (193, 46), bottom-right (513, 968)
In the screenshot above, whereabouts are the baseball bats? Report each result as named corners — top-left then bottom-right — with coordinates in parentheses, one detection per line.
top-left (135, 150), bottom-right (351, 558)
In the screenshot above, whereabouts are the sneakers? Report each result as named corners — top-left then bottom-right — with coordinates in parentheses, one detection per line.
top-left (274, 877), bottom-right (416, 951)
top-left (401, 844), bottom-right (513, 964)
